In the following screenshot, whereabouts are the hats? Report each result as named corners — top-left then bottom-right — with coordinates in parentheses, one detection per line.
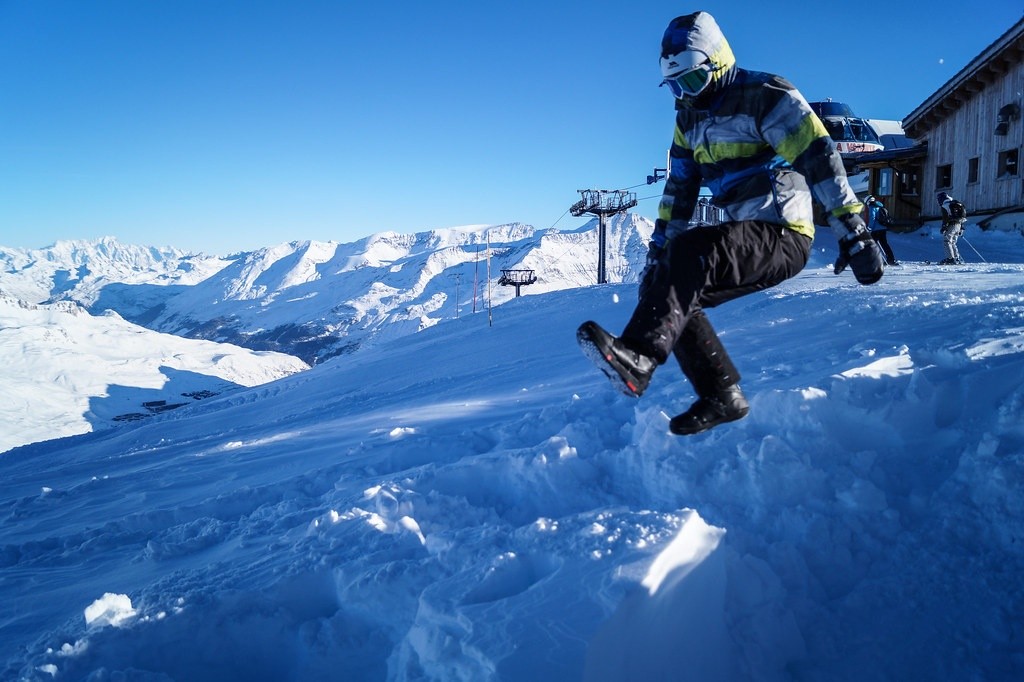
top-left (657, 50), bottom-right (707, 77)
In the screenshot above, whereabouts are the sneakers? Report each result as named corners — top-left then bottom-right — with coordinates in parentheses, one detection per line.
top-left (667, 382), bottom-right (746, 436)
top-left (576, 321), bottom-right (658, 398)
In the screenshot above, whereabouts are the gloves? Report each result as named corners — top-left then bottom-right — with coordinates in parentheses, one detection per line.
top-left (828, 209), bottom-right (884, 286)
top-left (633, 241), bottom-right (666, 302)
top-left (940, 225), bottom-right (947, 234)
top-left (958, 227), bottom-right (963, 237)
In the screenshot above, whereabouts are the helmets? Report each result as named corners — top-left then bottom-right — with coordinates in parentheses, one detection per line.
top-left (862, 194), bottom-right (875, 205)
top-left (937, 190), bottom-right (946, 204)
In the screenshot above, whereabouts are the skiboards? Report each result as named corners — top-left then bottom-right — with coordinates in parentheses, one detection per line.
top-left (923, 258), bottom-right (967, 267)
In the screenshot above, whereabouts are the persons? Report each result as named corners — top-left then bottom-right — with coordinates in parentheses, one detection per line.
top-left (937, 191), bottom-right (967, 266)
top-left (861, 193), bottom-right (902, 268)
top-left (576, 10), bottom-right (885, 437)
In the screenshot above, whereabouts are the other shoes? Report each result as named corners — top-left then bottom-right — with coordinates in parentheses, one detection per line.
top-left (883, 257), bottom-right (895, 265)
top-left (941, 256), bottom-right (963, 264)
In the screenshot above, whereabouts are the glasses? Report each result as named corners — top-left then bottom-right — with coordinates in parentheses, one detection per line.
top-left (660, 63), bottom-right (712, 100)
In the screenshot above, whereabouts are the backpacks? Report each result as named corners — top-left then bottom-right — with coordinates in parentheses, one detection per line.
top-left (948, 198), bottom-right (966, 221)
top-left (877, 203), bottom-right (894, 227)
